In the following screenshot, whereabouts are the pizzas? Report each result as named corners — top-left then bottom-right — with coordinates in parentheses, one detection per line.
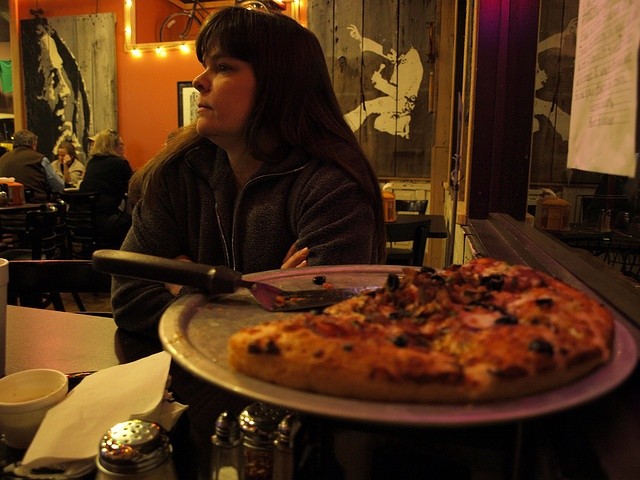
top-left (230, 259), bottom-right (613, 403)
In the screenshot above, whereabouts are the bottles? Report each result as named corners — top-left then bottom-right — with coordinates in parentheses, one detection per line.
top-left (209, 412), bottom-right (244, 480)
top-left (238, 402), bottom-right (301, 480)
top-left (95, 420), bottom-right (177, 480)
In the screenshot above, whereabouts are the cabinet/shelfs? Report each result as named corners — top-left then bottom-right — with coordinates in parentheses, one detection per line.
top-left (0, 0), bottom-right (24, 149)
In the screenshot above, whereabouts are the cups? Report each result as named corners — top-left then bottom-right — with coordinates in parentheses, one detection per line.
top-left (0, 369), bottom-right (69, 446)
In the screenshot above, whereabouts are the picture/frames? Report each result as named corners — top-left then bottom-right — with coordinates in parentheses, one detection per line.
top-left (177, 80), bottom-right (201, 127)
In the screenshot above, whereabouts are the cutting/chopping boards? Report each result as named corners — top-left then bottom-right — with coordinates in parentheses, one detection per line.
top-left (158, 264), bottom-right (638, 427)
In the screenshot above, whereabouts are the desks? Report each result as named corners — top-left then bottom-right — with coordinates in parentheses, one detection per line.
top-left (6, 302), bottom-right (121, 394)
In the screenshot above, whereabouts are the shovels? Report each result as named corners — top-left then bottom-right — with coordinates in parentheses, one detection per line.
top-left (93, 249), bottom-right (384, 312)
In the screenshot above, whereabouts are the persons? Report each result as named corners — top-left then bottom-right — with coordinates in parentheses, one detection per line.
top-left (26, 20), bottom-right (86, 156)
top-left (75, 129), bottom-right (136, 259)
top-left (0, 129), bottom-right (63, 200)
top-left (343, 22), bottom-right (424, 143)
top-left (50, 141), bottom-right (85, 186)
top-left (110, 7), bottom-right (387, 334)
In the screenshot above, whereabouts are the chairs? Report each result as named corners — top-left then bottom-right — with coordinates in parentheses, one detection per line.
top-left (59, 190), bottom-right (100, 260)
top-left (55, 199), bottom-right (87, 313)
top-left (396, 198), bottom-right (428, 213)
top-left (603, 240), bottom-right (640, 278)
top-left (560, 236), bottom-right (602, 257)
top-left (383, 217), bottom-right (432, 266)
top-left (5, 204), bottom-right (64, 311)
